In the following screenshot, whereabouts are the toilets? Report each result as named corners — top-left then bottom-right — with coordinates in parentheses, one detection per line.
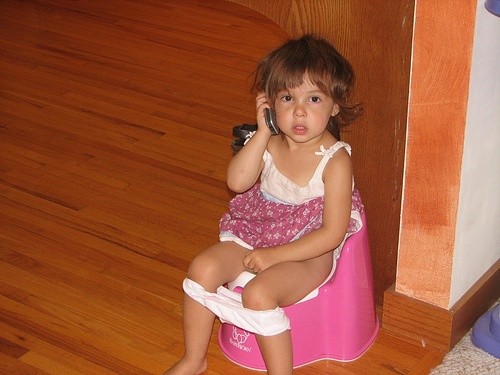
top-left (217, 209), bottom-right (379, 371)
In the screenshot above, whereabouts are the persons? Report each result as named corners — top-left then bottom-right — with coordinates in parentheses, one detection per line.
top-left (164, 32), bottom-right (363, 374)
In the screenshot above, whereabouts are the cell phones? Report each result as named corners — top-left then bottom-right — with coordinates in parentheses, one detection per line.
top-left (264, 107), bottom-right (279, 135)
